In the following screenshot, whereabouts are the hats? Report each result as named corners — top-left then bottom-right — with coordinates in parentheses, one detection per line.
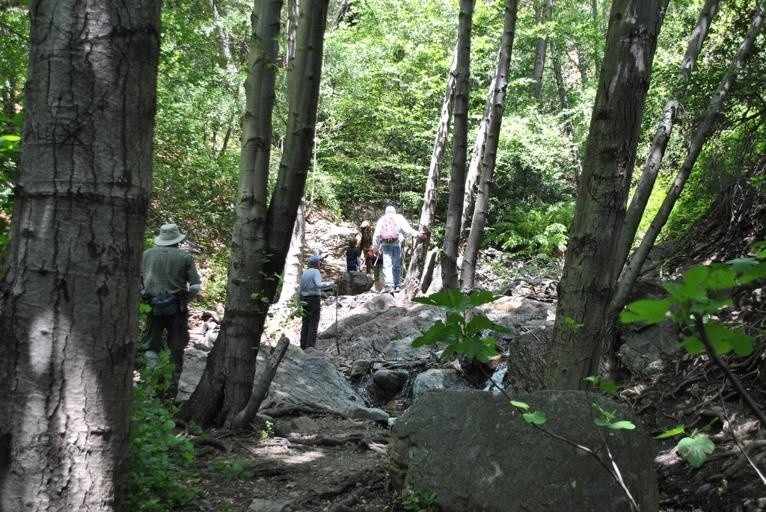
top-left (307, 255), bottom-right (322, 263)
top-left (152, 224), bottom-right (186, 246)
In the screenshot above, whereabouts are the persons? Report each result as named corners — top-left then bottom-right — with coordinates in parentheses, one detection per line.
top-left (358, 219), bottom-right (375, 274)
top-left (368, 206), bottom-right (427, 294)
top-left (141, 223), bottom-right (201, 401)
top-left (299, 254), bottom-right (334, 348)
top-left (346, 239), bottom-right (361, 273)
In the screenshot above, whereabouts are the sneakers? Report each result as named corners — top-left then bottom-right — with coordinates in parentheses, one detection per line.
top-left (381, 286), bottom-right (400, 293)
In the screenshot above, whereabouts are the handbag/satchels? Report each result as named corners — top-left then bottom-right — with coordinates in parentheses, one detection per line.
top-left (149, 294), bottom-right (178, 316)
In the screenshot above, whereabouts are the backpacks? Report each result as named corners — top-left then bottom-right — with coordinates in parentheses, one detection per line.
top-left (380, 214), bottom-right (400, 243)
